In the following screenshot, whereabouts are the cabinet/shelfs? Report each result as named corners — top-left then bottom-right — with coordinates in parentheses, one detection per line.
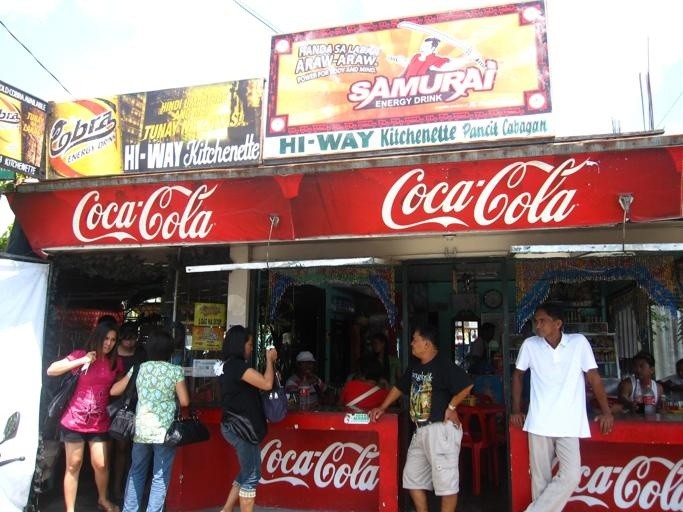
top-left (509, 282), bottom-right (621, 404)
top-left (180, 276), bottom-right (227, 402)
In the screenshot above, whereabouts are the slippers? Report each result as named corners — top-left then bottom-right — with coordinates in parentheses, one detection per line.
top-left (98, 500), bottom-right (121, 512)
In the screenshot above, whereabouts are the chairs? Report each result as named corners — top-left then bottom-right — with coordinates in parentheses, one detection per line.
top-left (455, 404), bottom-right (504, 493)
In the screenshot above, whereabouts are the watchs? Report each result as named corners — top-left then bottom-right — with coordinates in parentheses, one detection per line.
top-left (448, 401), bottom-right (460, 414)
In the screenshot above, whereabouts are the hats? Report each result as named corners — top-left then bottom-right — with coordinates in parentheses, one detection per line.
top-left (147, 335), bottom-right (174, 361)
top-left (296, 352), bottom-right (315, 361)
top-left (120, 322), bottom-right (138, 340)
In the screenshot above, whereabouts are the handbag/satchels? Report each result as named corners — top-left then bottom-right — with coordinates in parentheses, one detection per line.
top-left (164, 401), bottom-right (211, 447)
top-left (107, 360), bottom-right (145, 440)
top-left (48, 371), bottom-right (80, 419)
top-left (261, 362), bottom-right (288, 423)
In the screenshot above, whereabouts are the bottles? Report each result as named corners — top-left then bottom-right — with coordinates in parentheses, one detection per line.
top-left (299, 375), bottom-right (309, 412)
top-left (660, 394), bottom-right (666, 414)
top-left (643, 385), bottom-right (655, 417)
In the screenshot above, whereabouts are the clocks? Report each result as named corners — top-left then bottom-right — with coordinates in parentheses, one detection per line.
top-left (485, 289), bottom-right (503, 308)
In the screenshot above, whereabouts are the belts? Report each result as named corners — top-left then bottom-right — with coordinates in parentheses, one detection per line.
top-left (414, 421), bottom-right (430, 427)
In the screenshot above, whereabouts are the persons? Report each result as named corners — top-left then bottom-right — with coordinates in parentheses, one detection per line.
top-left (617, 350), bottom-right (664, 412)
top-left (288, 350), bottom-right (329, 411)
top-left (509, 299), bottom-right (614, 511)
top-left (368, 328), bottom-right (475, 512)
top-left (219, 324), bottom-right (279, 512)
top-left (110, 322), bottom-right (190, 512)
top-left (664, 357), bottom-right (683, 402)
top-left (116, 323), bottom-right (142, 374)
top-left (46, 321), bottom-right (120, 512)
top-left (459, 317), bottom-right (536, 384)
